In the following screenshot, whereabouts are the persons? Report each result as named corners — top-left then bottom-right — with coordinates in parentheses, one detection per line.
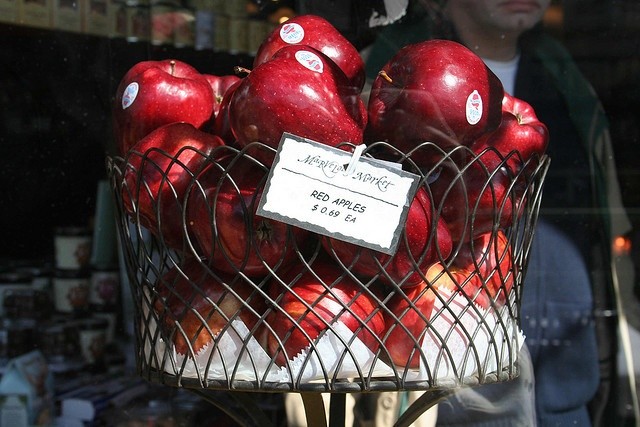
top-left (346, 1), bottom-right (633, 427)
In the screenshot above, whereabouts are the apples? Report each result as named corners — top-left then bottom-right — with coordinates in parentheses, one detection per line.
top-left (368, 40), bottom-right (505, 150)
top-left (316, 185), bottom-right (454, 289)
top-left (455, 227), bottom-right (515, 307)
top-left (202, 73), bottom-right (245, 146)
top-left (186, 170), bottom-right (306, 277)
top-left (264, 261), bottom-right (385, 368)
top-left (424, 167), bottom-right (529, 247)
top-left (465, 92), bottom-right (550, 174)
top-left (112, 59), bottom-right (214, 158)
top-left (382, 266), bottom-right (503, 369)
top-left (228, 44), bottom-right (369, 169)
top-left (153, 263), bottom-right (263, 359)
top-left (118, 120), bottom-right (227, 234)
top-left (252, 16), bottom-right (366, 98)
